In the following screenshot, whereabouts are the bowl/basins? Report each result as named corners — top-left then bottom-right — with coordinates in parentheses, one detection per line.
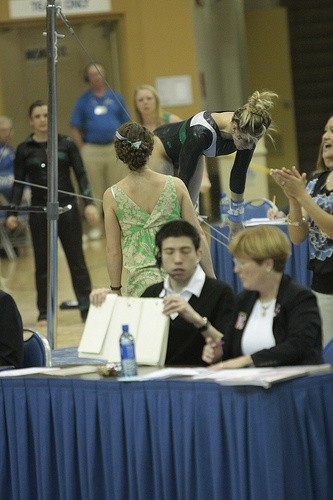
top-left (98, 365), bottom-right (122, 377)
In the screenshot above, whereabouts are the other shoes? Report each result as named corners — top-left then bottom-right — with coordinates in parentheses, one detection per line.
top-left (37, 313), bottom-right (48, 324)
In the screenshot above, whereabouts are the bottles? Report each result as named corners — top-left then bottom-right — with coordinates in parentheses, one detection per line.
top-left (219, 192), bottom-right (231, 225)
top-left (119, 325), bottom-right (138, 376)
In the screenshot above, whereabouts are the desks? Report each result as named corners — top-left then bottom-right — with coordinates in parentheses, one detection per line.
top-left (0, 363), bottom-right (333, 500)
top-left (210, 223), bottom-right (313, 292)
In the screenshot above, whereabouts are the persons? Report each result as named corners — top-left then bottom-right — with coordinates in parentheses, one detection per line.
top-left (0, 289), bottom-right (28, 369)
top-left (6, 100), bottom-right (99, 326)
top-left (269, 116), bottom-right (333, 295)
top-left (89, 220), bottom-right (236, 369)
top-left (149, 91), bottom-right (280, 241)
top-left (102, 122), bottom-right (217, 298)
top-left (267, 206), bottom-right (289, 221)
top-left (202, 225), bottom-right (323, 372)
top-left (133, 85), bottom-right (182, 132)
top-left (67, 64), bottom-right (137, 240)
top-left (0, 115), bottom-right (31, 259)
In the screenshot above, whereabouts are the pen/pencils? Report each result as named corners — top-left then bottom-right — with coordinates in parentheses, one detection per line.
top-left (272, 195), bottom-right (275, 222)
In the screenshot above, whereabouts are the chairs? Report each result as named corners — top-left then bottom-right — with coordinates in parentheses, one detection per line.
top-left (244, 199), bottom-right (278, 220)
top-left (23, 328), bottom-right (51, 367)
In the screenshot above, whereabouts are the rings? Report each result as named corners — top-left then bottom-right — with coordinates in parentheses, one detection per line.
top-left (281, 181), bottom-right (286, 186)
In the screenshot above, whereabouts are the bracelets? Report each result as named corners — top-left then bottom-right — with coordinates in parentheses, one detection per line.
top-left (287, 215), bottom-right (306, 226)
top-left (110, 285), bottom-right (122, 291)
top-left (197, 317), bottom-right (211, 332)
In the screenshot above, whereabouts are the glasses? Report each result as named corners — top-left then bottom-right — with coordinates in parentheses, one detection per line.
top-left (232, 257), bottom-right (248, 269)
